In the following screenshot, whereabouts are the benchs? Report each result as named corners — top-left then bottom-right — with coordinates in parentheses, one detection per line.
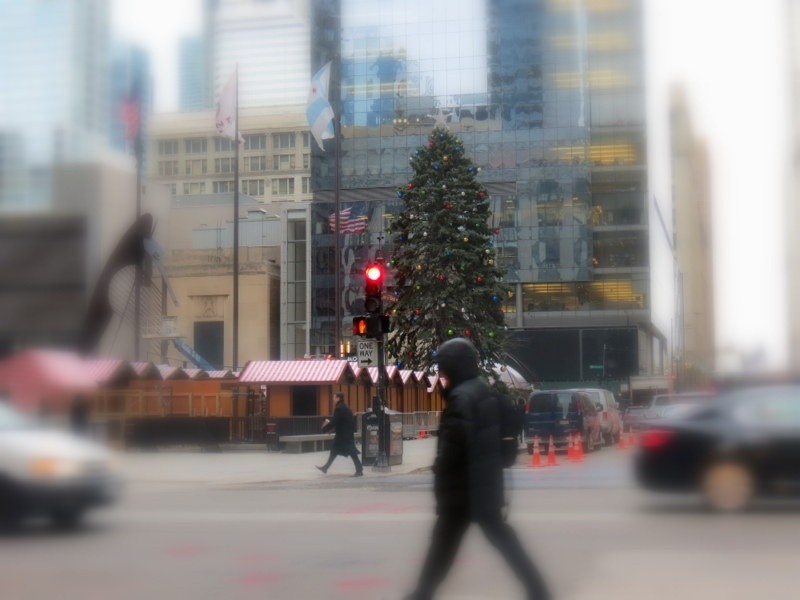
top-left (279, 431), bottom-right (362, 454)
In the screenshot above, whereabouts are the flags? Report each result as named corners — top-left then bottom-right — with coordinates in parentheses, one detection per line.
top-left (328, 202), bottom-right (367, 234)
top-left (305, 58), bottom-right (336, 152)
top-left (110, 54), bottom-right (142, 154)
top-left (215, 70), bottom-right (246, 144)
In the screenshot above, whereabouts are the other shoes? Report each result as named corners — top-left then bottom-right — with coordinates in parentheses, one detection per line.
top-left (354, 471), bottom-right (363, 477)
top-left (315, 465), bottom-right (327, 473)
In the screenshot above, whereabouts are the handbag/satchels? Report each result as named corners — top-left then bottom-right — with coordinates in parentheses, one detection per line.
top-left (498, 393), bottom-right (518, 467)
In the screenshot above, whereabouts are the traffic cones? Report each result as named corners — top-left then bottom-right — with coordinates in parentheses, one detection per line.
top-left (421, 420), bottom-right (427, 437)
top-left (528, 432), bottom-right (586, 467)
top-left (616, 424), bottom-right (635, 449)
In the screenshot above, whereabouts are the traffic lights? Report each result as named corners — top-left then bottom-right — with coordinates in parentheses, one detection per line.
top-left (352, 262), bottom-right (383, 338)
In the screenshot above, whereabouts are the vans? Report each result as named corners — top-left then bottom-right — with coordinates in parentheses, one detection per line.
top-left (525, 389), bottom-right (647, 455)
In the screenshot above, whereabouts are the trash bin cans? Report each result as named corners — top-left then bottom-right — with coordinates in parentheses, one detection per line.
top-left (361, 411), bottom-right (403, 467)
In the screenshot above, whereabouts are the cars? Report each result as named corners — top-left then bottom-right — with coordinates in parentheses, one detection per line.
top-left (634, 382), bottom-right (800, 514)
top-left (0, 401), bottom-right (121, 530)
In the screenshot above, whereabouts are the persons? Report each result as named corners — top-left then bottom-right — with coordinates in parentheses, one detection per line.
top-left (403, 339), bottom-right (551, 600)
top-left (316, 393), bottom-right (364, 477)
